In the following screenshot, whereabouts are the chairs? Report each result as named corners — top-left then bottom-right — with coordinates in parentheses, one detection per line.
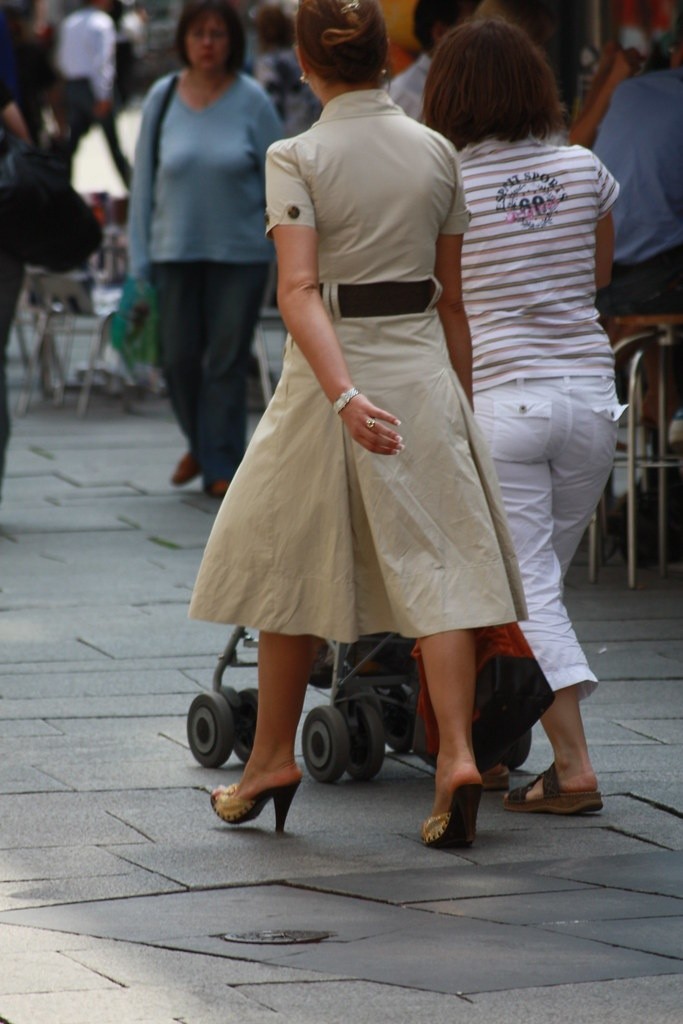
top-left (12, 231), bottom-right (126, 416)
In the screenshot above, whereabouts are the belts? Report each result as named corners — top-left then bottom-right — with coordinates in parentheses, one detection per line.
top-left (317, 279), bottom-right (436, 319)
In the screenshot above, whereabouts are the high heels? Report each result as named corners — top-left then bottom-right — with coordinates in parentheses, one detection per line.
top-left (209, 768), bottom-right (302, 832)
top-left (419, 781), bottom-right (483, 846)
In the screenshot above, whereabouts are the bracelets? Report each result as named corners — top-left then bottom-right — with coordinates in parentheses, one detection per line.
top-left (333, 387), bottom-right (360, 414)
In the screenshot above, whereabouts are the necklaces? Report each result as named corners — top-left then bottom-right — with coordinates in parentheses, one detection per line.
top-left (195, 77), bottom-right (223, 108)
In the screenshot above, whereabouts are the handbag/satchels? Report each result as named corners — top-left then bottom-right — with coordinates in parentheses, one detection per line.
top-left (409, 610), bottom-right (559, 754)
top-left (112, 280), bottom-right (160, 366)
top-left (0, 127), bottom-right (103, 275)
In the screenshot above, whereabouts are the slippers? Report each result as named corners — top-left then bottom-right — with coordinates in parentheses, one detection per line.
top-left (503, 760), bottom-right (603, 814)
top-left (483, 765), bottom-right (511, 793)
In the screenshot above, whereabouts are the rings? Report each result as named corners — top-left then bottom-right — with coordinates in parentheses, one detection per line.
top-left (366, 419), bottom-right (376, 429)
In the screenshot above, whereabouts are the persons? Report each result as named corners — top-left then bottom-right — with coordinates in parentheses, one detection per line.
top-left (242, 3), bottom-right (324, 133)
top-left (384, 0), bottom-right (483, 126)
top-left (126, 0), bottom-right (284, 498)
top-left (184, 0), bottom-right (628, 848)
top-left (0, 9), bottom-right (39, 503)
top-left (52, 0), bottom-right (133, 189)
top-left (567, 12), bottom-right (683, 476)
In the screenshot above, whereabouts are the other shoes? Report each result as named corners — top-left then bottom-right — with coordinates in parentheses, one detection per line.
top-left (172, 456), bottom-right (202, 484)
top-left (608, 496), bottom-right (648, 566)
top-left (207, 479), bottom-right (230, 497)
top-left (668, 419), bottom-right (683, 475)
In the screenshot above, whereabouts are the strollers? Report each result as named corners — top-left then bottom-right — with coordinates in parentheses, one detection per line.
top-left (185, 626), bottom-right (531, 786)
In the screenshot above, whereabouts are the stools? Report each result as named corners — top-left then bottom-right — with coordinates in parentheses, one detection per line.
top-left (588, 316), bottom-right (683, 590)
